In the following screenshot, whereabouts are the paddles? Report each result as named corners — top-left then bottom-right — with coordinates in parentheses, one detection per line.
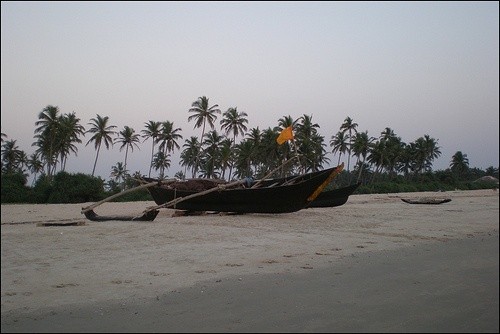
top-left (81, 177), bottom-right (249, 221)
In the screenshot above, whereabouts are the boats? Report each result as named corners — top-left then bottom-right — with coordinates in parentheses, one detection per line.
top-left (137, 161), bottom-right (345, 214)
top-left (306, 182), bottom-right (361, 209)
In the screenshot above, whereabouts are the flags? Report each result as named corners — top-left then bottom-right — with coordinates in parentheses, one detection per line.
top-left (276, 126), bottom-right (294, 144)
